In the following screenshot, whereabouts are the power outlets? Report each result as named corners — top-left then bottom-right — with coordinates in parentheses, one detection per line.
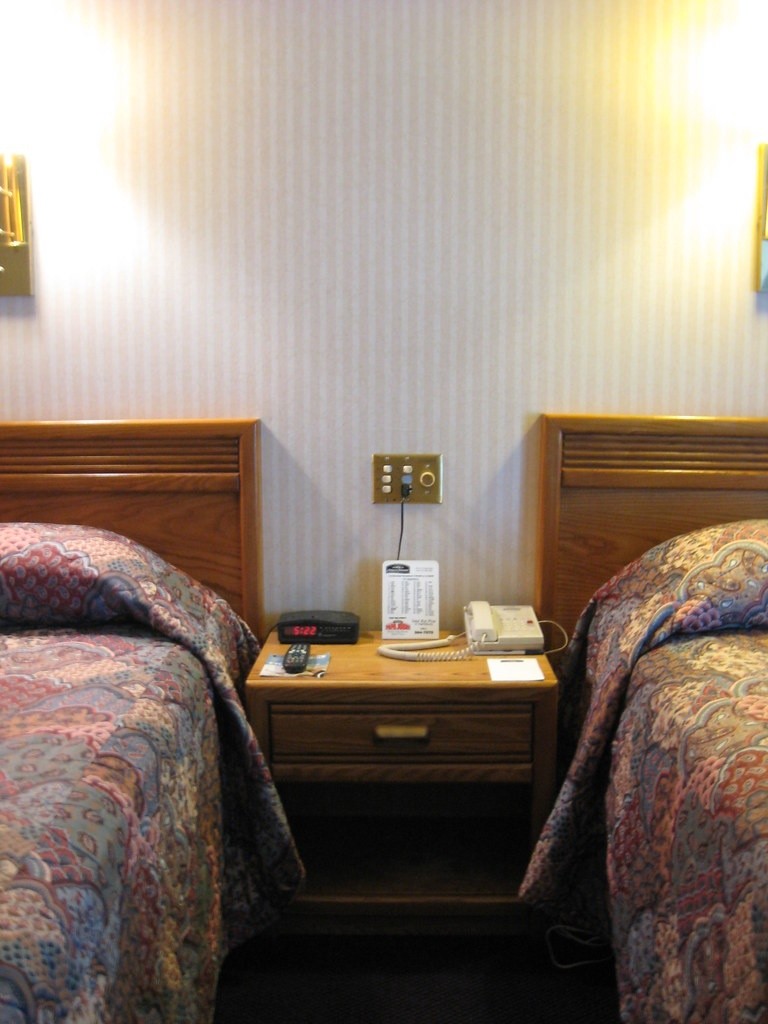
top-left (372, 454), bottom-right (443, 505)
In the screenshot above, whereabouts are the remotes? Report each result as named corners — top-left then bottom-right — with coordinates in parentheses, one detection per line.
top-left (283, 642), bottom-right (311, 674)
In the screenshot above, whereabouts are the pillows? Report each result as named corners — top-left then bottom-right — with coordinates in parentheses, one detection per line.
top-left (650, 518), bottom-right (768, 633)
top-left (0, 521), bottom-right (143, 632)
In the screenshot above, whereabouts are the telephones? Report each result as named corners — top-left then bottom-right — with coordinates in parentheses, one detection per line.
top-left (463, 600), bottom-right (545, 654)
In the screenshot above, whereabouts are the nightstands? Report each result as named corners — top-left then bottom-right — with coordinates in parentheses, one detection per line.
top-left (242, 627), bottom-right (560, 967)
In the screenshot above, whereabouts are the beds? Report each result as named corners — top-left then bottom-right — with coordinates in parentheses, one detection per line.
top-left (0, 417), bottom-right (308, 1024)
top-left (518, 414), bottom-right (768, 1024)
